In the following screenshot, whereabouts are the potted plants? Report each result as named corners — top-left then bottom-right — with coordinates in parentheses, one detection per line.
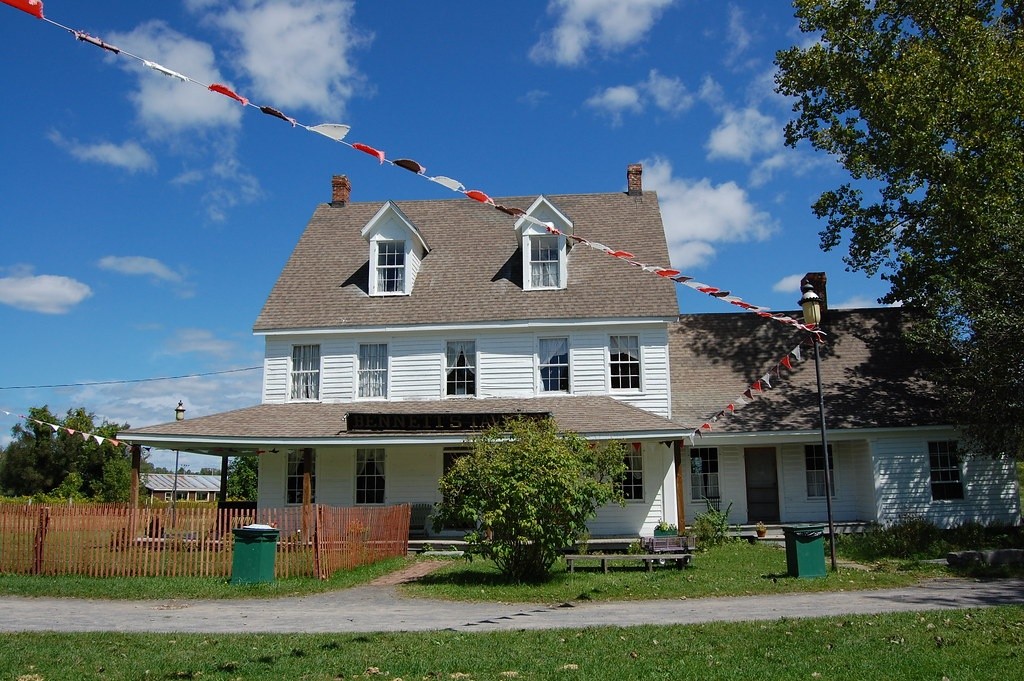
top-left (756, 521), bottom-right (767, 536)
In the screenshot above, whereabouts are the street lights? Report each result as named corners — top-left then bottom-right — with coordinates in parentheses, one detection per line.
top-left (798, 279), bottom-right (837, 572)
top-left (170, 399), bottom-right (186, 527)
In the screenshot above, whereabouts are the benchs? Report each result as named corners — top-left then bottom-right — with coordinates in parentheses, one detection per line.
top-left (566, 554), bottom-right (694, 575)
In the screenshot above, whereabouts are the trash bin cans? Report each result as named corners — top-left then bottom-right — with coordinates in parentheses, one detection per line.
top-left (231, 523), bottom-right (280, 585)
top-left (781, 524), bottom-right (828, 579)
